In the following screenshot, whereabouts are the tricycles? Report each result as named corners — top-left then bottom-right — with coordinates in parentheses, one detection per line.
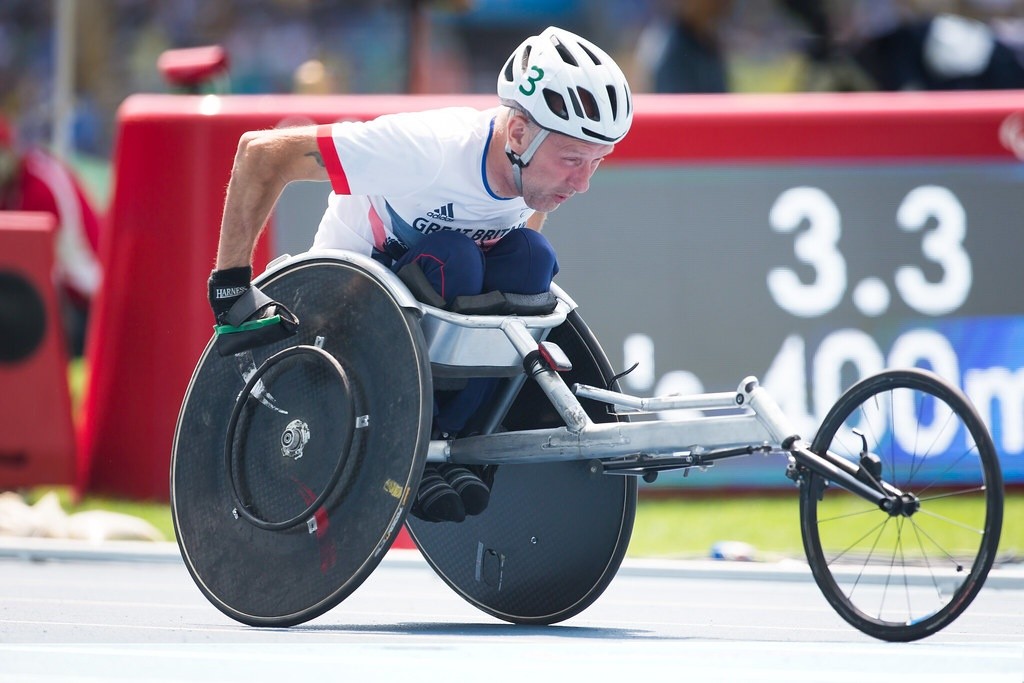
top-left (165, 248), bottom-right (1006, 641)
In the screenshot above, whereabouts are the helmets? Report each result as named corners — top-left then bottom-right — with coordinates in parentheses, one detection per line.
top-left (497, 26), bottom-right (634, 145)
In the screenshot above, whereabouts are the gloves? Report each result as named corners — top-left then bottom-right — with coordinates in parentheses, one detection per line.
top-left (208, 265), bottom-right (299, 357)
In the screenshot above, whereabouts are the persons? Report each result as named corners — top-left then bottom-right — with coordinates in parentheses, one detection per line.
top-left (205, 25), bottom-right (636, 526)
top-left (0, 92), bottom-right (112, 362)
top-left (623, 0), bottom-right (1024, 95)
